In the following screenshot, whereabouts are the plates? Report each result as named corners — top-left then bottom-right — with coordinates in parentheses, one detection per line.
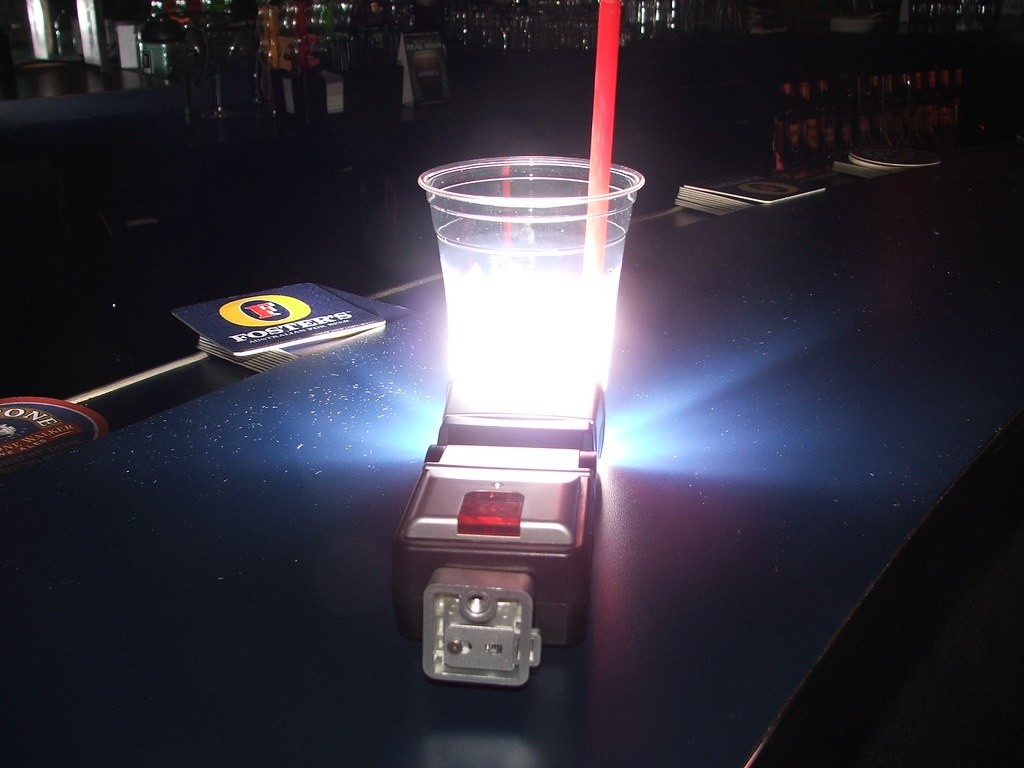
top-left (849, 146), bottom-right (941, 172)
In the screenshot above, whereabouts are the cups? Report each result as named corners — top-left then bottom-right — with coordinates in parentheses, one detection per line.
top-left (418, 157), bottom-right (648, 410)
top-left (442, 0), bottom-right (679, 52)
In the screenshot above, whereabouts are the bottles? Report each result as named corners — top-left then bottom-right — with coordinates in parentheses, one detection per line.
top-left (135, 0), bottom-right (415, 124)
top-left (772, 68), bottom-right (967, 169)
